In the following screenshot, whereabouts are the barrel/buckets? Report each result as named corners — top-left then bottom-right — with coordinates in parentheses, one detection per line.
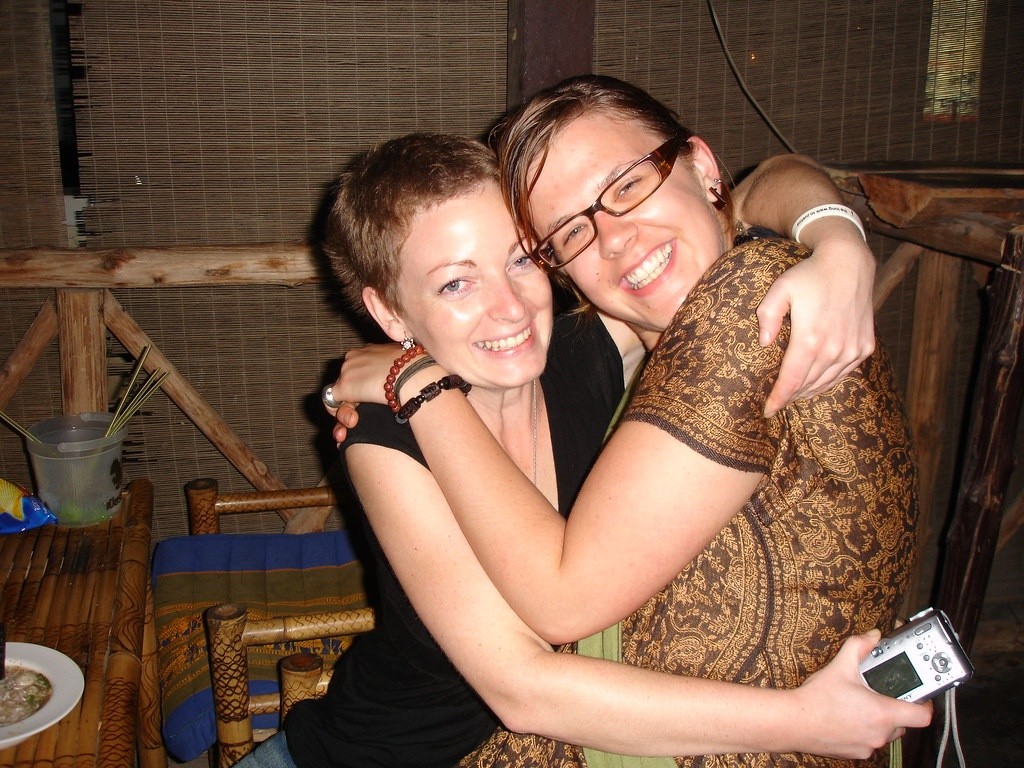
top-left (25, 413), bottom-right (128, 528)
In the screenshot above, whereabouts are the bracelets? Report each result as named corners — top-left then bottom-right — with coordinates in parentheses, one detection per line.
top-left (383, 345), bottom-right (472, 424)
top-left (791, 203), bottom-right (866, 244)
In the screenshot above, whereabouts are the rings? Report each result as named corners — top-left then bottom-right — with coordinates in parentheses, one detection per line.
top-left (322, 383), bottom-right (343, 408)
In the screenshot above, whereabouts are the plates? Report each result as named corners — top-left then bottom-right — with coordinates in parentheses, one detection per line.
top-left (0, 639), bottom-right (85, 748)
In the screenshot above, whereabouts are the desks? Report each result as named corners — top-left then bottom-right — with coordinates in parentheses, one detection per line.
top-left (0, 479), bottom-right (163, 768)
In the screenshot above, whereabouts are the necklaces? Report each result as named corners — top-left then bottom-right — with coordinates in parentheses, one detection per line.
top-left (533, 378), bottom-right (537, 486)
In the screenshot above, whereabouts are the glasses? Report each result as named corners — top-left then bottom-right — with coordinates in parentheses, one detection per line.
top-left (528, 136), bottom-right (682, 271)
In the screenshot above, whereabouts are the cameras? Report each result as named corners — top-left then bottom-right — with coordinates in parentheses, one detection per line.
top-left (859, 606), bottom-right (974, 706)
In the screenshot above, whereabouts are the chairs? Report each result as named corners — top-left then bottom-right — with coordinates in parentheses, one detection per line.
top-left (151, 476), bottom-right (376, 768)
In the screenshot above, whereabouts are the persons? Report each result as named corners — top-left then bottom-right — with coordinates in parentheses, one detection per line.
top-left (321, 75), bottom-right (921, 768)
top-left (231, 130), bottom-right (934, 768)
